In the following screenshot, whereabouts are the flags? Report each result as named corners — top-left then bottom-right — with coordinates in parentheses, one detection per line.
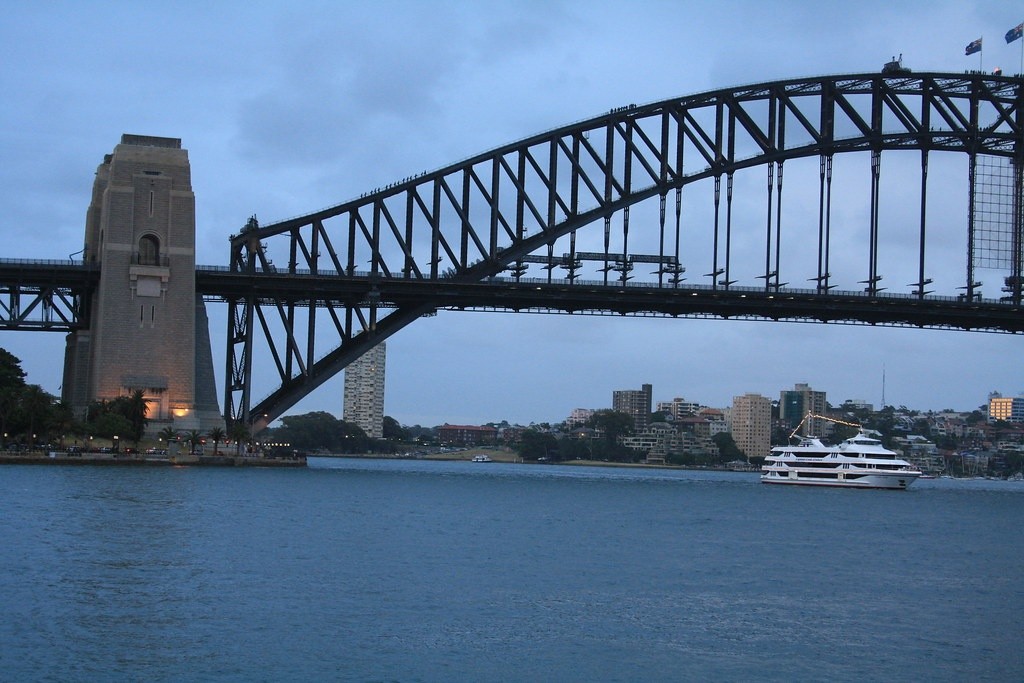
top-left (1005, 24), bottom-right (1022, 45)
top-left (964, 38), bottom-right (982, 56)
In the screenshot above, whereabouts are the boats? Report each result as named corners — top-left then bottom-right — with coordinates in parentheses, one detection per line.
top-left (920, 474), bottom-right (1024, 483)
top-left (760, 410), bottom-right (923, 489)
top-left (472, 455), bottom-right (493, 463)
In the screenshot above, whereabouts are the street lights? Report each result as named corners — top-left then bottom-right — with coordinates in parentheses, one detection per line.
top-left (251, 413), bottom-right (268, 456)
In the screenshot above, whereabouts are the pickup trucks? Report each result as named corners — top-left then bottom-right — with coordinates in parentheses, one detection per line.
top-left (100, 446), bottom-right (110, 453)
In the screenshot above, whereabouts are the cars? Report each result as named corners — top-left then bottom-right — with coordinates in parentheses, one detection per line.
top-left (537, 457), bottom-right (548, 462)
top-left (125, 447), bottom-right (167, 455)
top-left (66, 446), bottom-right (81, 453)
top-left (191, 449), bottom-right (204, 455)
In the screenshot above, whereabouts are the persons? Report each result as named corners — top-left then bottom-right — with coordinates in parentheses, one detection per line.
top-left (991, 72), bottom-right (998, 75)
top-left (965, 69), bottom-right (986, 74)
top-left (610, 103), bottom-right (636, 114)
top-left (360, 169), bottom-right (427, 199)
top-left (931, 126), bottom-right (934, 131)
top-left (940, 127), bottom-right (941, 131)
top-left (1014, 72), bottom-right (1024, 78)
top-left (980, 127), bottom-right (988, 132)
